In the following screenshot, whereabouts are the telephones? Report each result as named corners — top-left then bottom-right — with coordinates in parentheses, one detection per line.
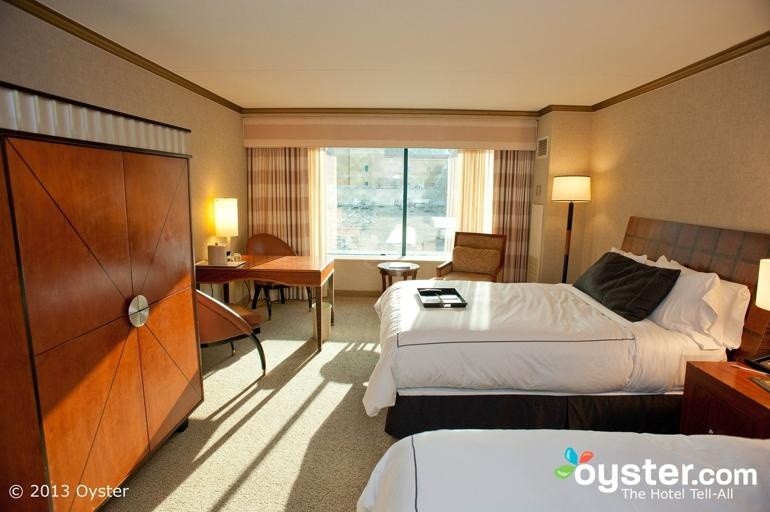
top-left (744, 351), bottom-right (770, 374)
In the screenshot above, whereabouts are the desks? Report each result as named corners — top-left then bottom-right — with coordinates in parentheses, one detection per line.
top-left (377, 262), bottom-right (421, 292)
top-left (195, 255), bottom-right (335, 352)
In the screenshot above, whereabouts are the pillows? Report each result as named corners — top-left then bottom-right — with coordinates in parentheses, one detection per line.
top-left (610, 247), bottom-right (751, 351)
top-left (452, 247), bottom-right (501, 275)
top-left (572, 252), bottom-right (681, 322)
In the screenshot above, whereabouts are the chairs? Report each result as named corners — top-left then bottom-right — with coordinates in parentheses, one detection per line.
top-left (247, 233), bottom-right (312, 321)
top-left (196, 289), bottom-right (266, 376)
top-left (429, 232), bottom-right (507, 282)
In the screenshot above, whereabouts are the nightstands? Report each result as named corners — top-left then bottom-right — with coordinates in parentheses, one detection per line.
top-left (681, 361), bottom-right (770, 439)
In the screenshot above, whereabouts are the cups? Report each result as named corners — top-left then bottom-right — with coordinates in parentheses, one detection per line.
top-left (233, 254), bottom-right (242, 263)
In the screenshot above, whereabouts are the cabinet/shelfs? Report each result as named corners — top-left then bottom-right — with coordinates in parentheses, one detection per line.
top-left (0, 127), bottom-right (204, 512)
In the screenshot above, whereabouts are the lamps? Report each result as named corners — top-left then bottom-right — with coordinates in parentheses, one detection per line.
top-left (215, 198), bottom-right (239, 256)
top-left (552, 175), bottom-right (592, 283)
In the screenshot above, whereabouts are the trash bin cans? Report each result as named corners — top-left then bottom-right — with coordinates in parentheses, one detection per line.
top-left (311, 302), bottom-right (333, 341)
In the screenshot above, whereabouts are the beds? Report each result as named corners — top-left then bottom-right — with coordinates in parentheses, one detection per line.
top-left (356, 429), bottom-right (770, 512)
top-left (362, 216), bottom-right (770, 439)
